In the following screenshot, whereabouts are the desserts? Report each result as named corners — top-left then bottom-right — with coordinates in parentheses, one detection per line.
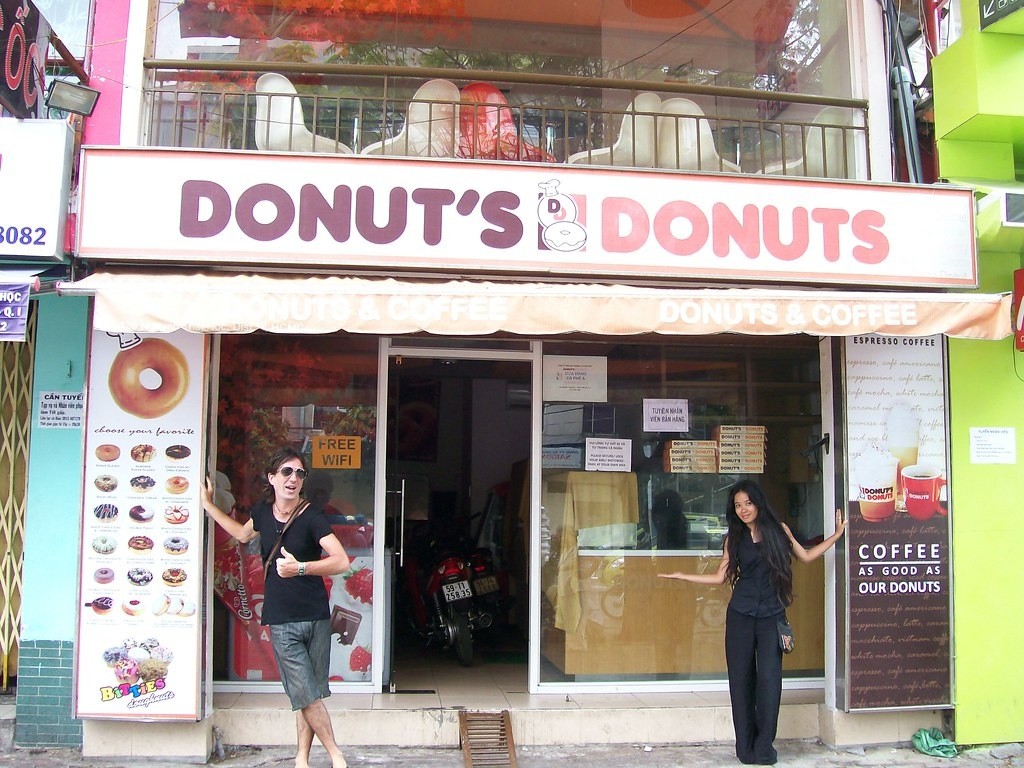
top-left (104, 637), bottom-right (173, 684)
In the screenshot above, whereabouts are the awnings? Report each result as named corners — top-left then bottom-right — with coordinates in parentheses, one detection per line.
top-left (55, 265), bottom-right (1016, 343)
top-left (0, 266), bottom-right (50, 342)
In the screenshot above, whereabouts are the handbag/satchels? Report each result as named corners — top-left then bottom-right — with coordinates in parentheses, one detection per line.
top-left (775, 614), bottom-right (795, 654)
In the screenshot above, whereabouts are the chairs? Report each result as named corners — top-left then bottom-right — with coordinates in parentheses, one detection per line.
top-left (563, 93), bottom-right (661, 168)
top-left (362, 79), bottom-right (460, 159)
top-left (662, 98), bottom-right (746, 173)
top-left (460, 82), bottom-right (557, 163)
top-left (756, 106), bottom-right (856, 179)
top-left (254, 72), bottom-right (353, 154)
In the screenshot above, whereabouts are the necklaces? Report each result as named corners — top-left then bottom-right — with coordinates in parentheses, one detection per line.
top-left (273, 503), bottom-right (291, 515)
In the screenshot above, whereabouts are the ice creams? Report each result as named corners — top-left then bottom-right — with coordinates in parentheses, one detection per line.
top-left (207, 472), bottom-right (252, 631)
top-left (887, 407), bottom-right (918, 499)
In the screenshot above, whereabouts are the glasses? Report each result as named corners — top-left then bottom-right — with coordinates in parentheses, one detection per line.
top-left (276, 467), bottom-right (307, 480)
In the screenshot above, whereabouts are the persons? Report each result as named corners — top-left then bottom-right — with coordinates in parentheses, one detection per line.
top-left (656, 480), bottom-right (848, 765)
top-left (198, 449), bottom-right (350, 768)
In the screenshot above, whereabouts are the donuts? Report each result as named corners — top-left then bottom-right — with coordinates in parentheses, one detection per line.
top-left (93, 445), bottom-right (196, 618)
top-left (109, 338), bottom-right (189, 419)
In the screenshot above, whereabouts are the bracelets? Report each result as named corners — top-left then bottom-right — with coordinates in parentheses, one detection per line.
top-left (297, 562), bottom-right (305, 577)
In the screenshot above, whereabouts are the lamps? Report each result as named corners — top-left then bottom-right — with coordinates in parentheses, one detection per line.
top-left (44, 78), bottom-right (101, 117)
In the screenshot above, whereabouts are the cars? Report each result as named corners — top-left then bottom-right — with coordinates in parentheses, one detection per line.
top-left (595, 512), bottom-right (723, 553)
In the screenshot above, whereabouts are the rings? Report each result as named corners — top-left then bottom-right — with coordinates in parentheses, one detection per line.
top-left (837, 517), bottom-right (840, 520)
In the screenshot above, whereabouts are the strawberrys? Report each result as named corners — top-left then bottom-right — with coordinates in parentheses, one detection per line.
top-left (350, 645), bottom-right (372, 673)
top-left (344, 566), bottom-right (373, 606)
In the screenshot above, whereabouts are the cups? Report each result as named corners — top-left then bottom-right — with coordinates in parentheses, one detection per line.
top-left (890, 447), bottom-right (918, 512)
top-left (858, 464), bottom-right (897, 522)
top-left (901, 465), bottom-right (948, 521)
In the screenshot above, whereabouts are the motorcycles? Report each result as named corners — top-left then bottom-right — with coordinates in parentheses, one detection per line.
top-left (468, 546), bottom-right (505, 655)
top-left (404, 511), bottom-right (492, 667)
